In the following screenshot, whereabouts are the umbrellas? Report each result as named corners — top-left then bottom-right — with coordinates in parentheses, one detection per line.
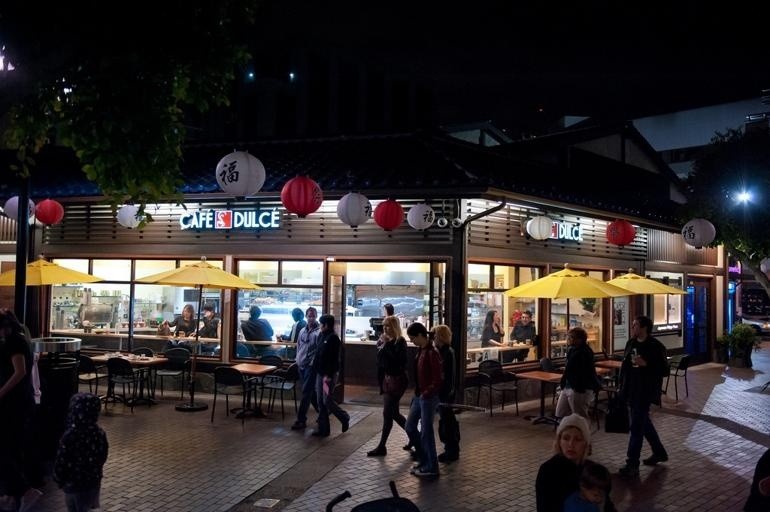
top-left (502, 263), bottom-right (637, 353)
top-left (132, 256), bottom-right (266, 406)
top-left (606, 268), bottom-right (691, 314)
top-left (0, 255), bottom-right (105, 337)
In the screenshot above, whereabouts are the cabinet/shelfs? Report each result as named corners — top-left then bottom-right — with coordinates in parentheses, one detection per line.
top-left (468, 293), bottom-right (487, 342)
top-left (551, 303), bottom-right (586, 330)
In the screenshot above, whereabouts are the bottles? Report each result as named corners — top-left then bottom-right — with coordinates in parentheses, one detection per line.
top-left (64, 297), bottom-right (70, 305)
top-left (88, 327), bottom-right (91, 333)
top-left (58, 297), bottom-right (64, 305)
top-left (53, 297), bottom-right (58, 305)
top-left (106, 323), bottom-right (110, 334)
top-left (115, 323), bottom-right (119, 334)
top-left (84, 326), bottom-right (87, 333)
top-left (88, 288), bottom-right (91, 305)
top-left (83, 288), bottom-right (87, 305)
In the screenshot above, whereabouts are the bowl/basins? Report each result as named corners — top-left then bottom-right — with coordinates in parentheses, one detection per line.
top-left (360, 337), bottom-right (367, 342)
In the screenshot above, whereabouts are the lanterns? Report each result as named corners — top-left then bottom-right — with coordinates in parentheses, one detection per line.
top-left (374, 200), bottom-right (404, 231)
top-left (607, 220), bottom-right (635, 246)
top-left (117, 205), bottom-right (142, 228)
top-left (281, 173), bottom-right (324, 218)
top-left (35, 199), bottom-right (64, 225)
top-left (5, 196), bottom-right (35, 219)
top-left (681, 218), bottom-right (716, 249)
top-left (406, 204), bottom-right (435, 231)
top-left (337, 192), bottom-right (372, 227)
top-left (215, 148), bottom-right (266, 201)
top-left (526, 216), bottom-right (553, 240)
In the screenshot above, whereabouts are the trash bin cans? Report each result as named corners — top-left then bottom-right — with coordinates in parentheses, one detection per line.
top-left (30, 337), bottom-right (82, 402)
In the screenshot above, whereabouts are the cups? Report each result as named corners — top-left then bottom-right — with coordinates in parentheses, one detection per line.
top-left (121, 323), bottom-right (126, 328)
top-left (526, 339), bottom-right (530, 345)
top-left (133, 323), bottom-right (138, 327)
top-left (179, 331), bottom-right (185, 338)
top-left (139, 323), bottom-right (144, 327)
top-left (508, 342), bottom-right (513, 347)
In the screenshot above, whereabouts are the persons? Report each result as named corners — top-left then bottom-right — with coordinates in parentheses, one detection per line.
top-left (480, 310), bottom-right (513, 363)
top-left (555, 327), bottom-right (604, 418)
top-left (161, 305), bottom-right (221, 339)
top-left (277, 306), bottom-right (350, 437)
top-left (616, 315), bottom-right (670, 477)
top-left (510, 311), bottom-right (535, 361)
top-left (742, 448), bottom-right (770, 512)
top-left (367, 304), bottom-right (459, 475)
top-left (241, 305), bottom-right (273, 341)
top-left (563, 464), bottom-right (612, 512)
top-left (535, 413), bottom-right (618, 512)
top-left (51, 391), bottom-right (108, 512)
top-left (0, 308), bottom-right (44, 512)
top-left (21, 324), bottom-right (48, 495)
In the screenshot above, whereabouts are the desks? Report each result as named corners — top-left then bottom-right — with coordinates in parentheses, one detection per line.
top-left (515, 370), bottom-right (565, 425)
top-left (559, 367), bottom-right (611, 419)
top-left (595, 360), bottom-right (623, 396)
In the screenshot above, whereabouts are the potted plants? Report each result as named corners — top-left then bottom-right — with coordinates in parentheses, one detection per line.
top-left (717, 324), bottom-right (762, 368)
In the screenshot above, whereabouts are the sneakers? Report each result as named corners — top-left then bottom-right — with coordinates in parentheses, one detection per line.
top-left (0, 495), bottom-right (16, 511)
top-left (410, 464), bottom-right (419, 473)
top-left (618, 465), bottom-right (640, 477)
top-left (416, 468), bottom-right (439, 475)
top-left (642, 454), bottom-right (669, 466)
top-left (18, 489), bottom-right (43, 511)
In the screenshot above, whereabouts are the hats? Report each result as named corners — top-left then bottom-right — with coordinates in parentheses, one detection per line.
top-left (556, 413), bottom-right (591, 445)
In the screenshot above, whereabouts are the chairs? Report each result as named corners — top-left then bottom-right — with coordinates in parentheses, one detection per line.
top-left (211, 355), bottom-right (298, 424)
top-left (665, 354), bottom-right (691, 400)
top-left (78, 347), bottom-right (191, 414)
top-left (477, 360), bottom-right (519, 416)
top-left (603, 348), bottom-right (619, 387)
top-left (540, 357), bottom-right (560, 404)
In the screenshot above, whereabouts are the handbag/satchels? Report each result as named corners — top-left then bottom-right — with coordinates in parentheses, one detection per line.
top-left (605, 394), bottom-right (630, 434)
top-left (157, 320), bottom-right (172, 336)
top-left (383, 375), bottom-right (401, 392)
